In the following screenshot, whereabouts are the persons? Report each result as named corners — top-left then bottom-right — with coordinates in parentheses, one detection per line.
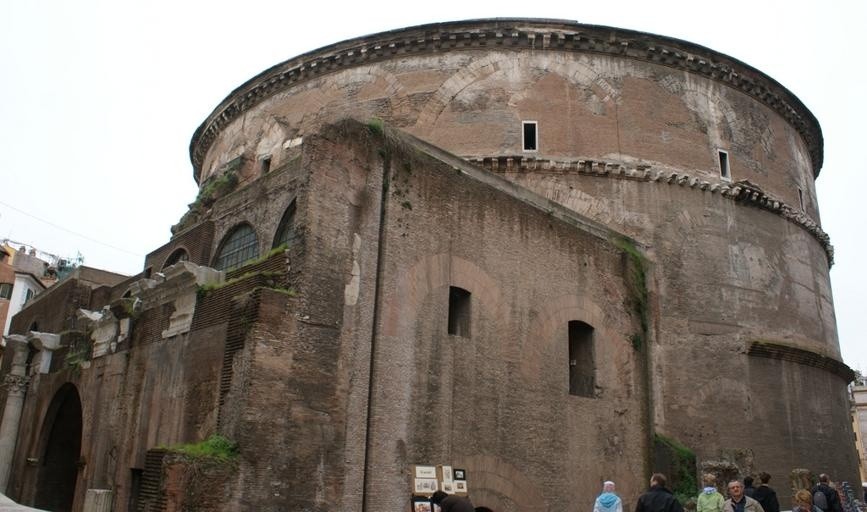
top-left (430, 491), bottom-right (474, 511)
top-left (592, 480), bottom-right (622, 512)
top-left (811, 473), bottom-right (844, 512)
top-left (695, 472), bottom-right (724, 511)
top-left (752, 472), bottom-right (780, 511)
top-left (723, 479), bottom-right (764, 512)
top-left (635, 471), bottom-right (685, 511)
top-left (792, 489), bottom-right (823, 512)
top-left (742, 476), bottom-right (756, 498)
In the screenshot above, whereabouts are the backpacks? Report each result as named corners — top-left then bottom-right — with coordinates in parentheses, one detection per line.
top-left (813, 490), bottom-right (830, 512)
